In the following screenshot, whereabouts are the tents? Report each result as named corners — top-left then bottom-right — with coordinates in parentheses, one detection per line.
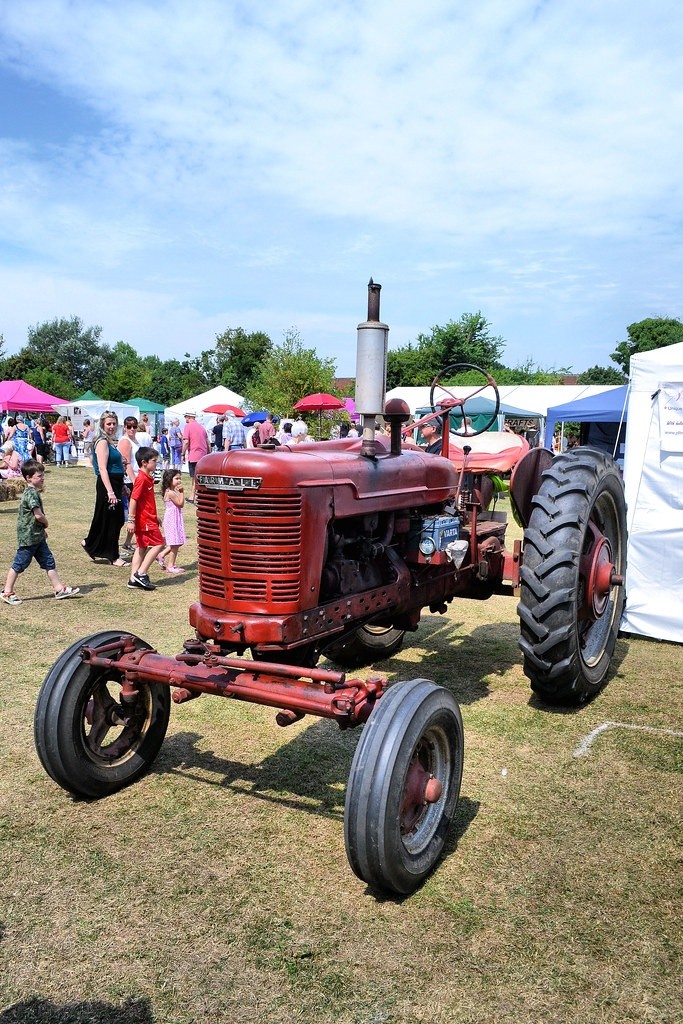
top-left (385, 341), bottom-right (683, 646)
top-left (0, 380), bottom-right (267, 442)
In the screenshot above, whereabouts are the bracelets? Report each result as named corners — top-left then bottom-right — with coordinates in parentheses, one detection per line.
top-left (128, 514), bottom-right (136, 523)
top-left (181, 453), bottom-right (185, 456)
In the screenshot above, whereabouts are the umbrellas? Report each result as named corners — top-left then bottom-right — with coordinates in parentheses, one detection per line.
top-left (293, 392), bottom-right (346, 442)
top-left (240, 410), bottom-right (278, 427)
top-left (203, 404), bottom-right (247, 417)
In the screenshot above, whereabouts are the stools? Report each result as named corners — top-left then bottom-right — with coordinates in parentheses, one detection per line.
top-left (443, 425), bottom-right (530, 473)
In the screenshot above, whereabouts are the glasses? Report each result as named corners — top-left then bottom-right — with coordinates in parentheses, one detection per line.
top-left (420, 424), bottom-right (433, 428)
top-left (127, 425), bottom-right (137, 429)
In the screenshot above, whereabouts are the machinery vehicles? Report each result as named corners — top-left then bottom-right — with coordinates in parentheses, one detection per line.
top-left (33, 277), bottom-right (628, 895)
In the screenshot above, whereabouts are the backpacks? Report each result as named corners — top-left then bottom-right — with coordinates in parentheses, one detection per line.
top-left (252, 430), bottom-right (260, 447)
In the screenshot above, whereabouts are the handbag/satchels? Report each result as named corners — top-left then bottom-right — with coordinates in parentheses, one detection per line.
top-left (71, 439), bottom-right (78, 458)
top-left (27, 435), bottom-right (35, 451)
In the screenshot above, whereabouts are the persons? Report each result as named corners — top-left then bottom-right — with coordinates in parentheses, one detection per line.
top-left (117, 416), bottom-right (143, 493)
top-left (155, 468), bottom-right (185, 574)
top-left (180, 407), bottom-right (211, 504)
top-left (80, 410), bottom-right (131, 566)
top-left (119, 457), bottom-right (135, 551)
top-left (126, 446), bottom-right (165, 591)
top-left (0, 416), bottom-right (581, 487)
top-left (0, 460), bottom-right (81, 606)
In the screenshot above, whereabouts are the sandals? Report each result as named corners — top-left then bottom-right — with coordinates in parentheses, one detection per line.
top-left (122, 544), bottom-right (135, 551)
top-left (81, 539), bottom-right (96, 562)
top-left (109, 559), bottom-right (130, 567)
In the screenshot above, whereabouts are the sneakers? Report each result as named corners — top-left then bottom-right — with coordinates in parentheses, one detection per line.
top-left (128, 578), bottom-right (145, 588)
top-left (0, 590), bottom-right (23, 605)
top-left (55, 587), bottom-right (80, 600)
top-left (133, 570), bottom-right (155, 589)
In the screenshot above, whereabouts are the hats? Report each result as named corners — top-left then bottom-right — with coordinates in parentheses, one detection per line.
top-left (413, 413), bottom-right (443, 430)
top-left (183, 410), bottom-right (197, 417)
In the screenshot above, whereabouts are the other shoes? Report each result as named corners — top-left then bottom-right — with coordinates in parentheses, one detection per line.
top-left (155, 554), bottom-right (167, 568)
top-left (166, 566), bottom-right (185, 573)
